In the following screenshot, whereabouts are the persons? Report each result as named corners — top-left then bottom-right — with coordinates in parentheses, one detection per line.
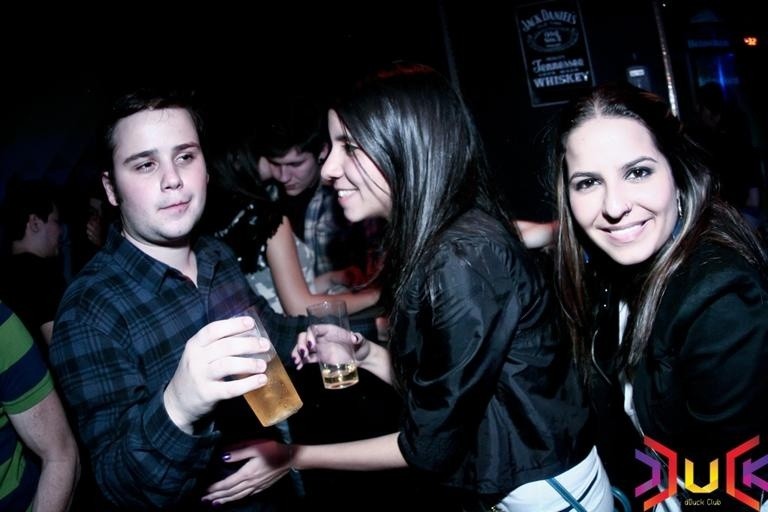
top-left (0, 63), bottom-right (768, 512)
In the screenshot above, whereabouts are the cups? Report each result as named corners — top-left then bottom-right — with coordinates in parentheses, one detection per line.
top-left (221, 304), bottom-right (306, 427)
top-left (308, 300), bottom-right (359, 391)
